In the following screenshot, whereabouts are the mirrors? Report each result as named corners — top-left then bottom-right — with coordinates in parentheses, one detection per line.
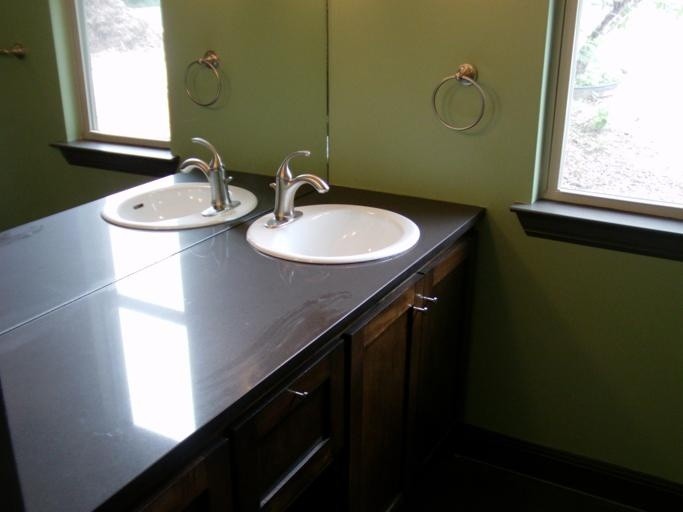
top-left (1, 2), bottom-right (327, 334)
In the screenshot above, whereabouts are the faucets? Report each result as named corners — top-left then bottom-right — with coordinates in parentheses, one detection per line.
top-left (179, 137), bottom-right (233, 211)
top-left (265, 151), bottom-right (331, 228)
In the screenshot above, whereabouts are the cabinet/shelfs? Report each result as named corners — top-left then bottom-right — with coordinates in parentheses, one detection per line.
top-left (142, 223), bottom-right (479, 512)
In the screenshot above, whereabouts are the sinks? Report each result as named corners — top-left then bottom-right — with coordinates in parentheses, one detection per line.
top-left (102, 181), bottom-right (257, 230)
top-left (246, 203), bottom-right (421, 266)
